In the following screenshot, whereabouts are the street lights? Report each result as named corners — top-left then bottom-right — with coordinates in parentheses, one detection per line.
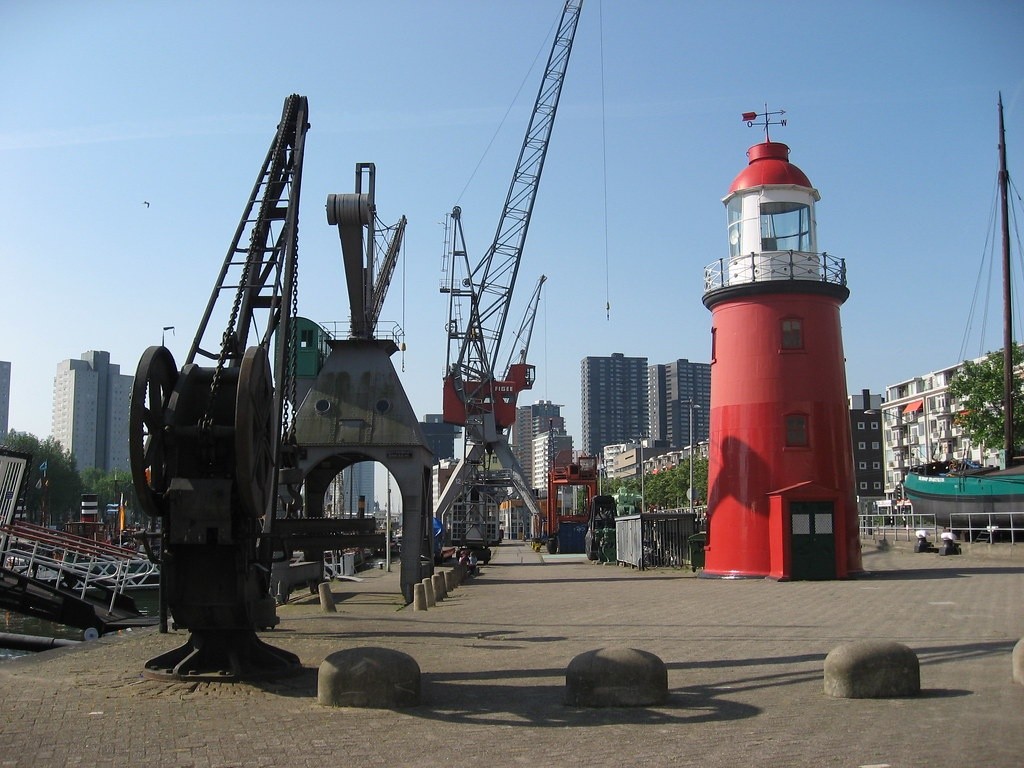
top-left (685, 398), bottom-right (702, 514)
top-left (865, 408), bottom-right (942, 470)
top-left (638, 432), bottom-right (645, 514)
top-left (160, 325), bottom-right (175, 346)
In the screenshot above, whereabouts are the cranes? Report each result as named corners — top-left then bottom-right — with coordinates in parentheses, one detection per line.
top-left (280, 161), bottom-right (430, 604)
top-left (133, 93), bottom-right (303, 679)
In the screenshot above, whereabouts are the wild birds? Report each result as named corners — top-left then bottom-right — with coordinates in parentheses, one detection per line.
top-left (140, 200), bottom-right (151, 209)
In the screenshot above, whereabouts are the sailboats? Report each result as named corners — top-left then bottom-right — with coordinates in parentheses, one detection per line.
top-left (899, 90), bottom-right (1023, 540)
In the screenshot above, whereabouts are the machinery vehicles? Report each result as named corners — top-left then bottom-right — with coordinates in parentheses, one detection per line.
top-left (432, 0), bottom-right (607, 571)
